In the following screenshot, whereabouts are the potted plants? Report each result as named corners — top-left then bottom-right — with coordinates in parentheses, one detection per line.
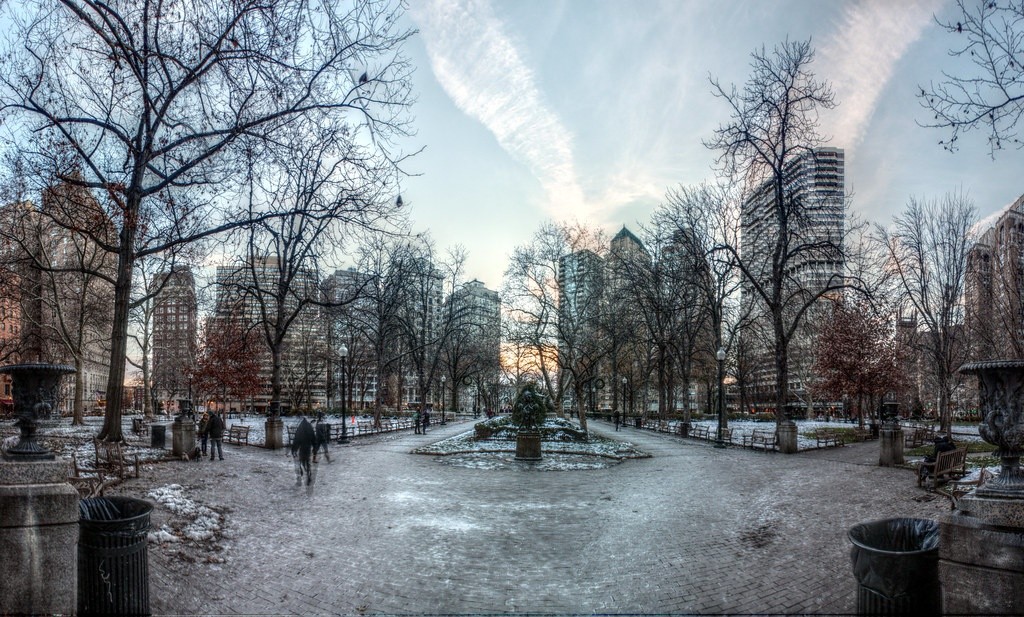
top-left (512, 379), bottom-right (548, 462)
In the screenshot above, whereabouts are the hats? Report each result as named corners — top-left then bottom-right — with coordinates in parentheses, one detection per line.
top-left (203, 413), bottom-right (208, 417)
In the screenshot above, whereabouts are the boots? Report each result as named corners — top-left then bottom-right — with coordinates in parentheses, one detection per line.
top-left (312, 454), bottom-right (318, 464)
top-left (325, 453), bottom-right (335, 465)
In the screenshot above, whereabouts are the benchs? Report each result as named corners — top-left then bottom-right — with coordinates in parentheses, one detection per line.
top-left (815, 428), bottom-right (845, 449)
top-left (221, 424), bottom-right (250, 446)
top-left (626, 417), bottom-right (682, 435)
top-left (853, 426), bottom-right (874, 442)
top-left (918, 443), bottom-right (969, 488)
top-left (135, 420), bottom-right (150, 436)
top-left (707, 428), bottom-right (734, 446)
top-left (69, 435), bottom-right (141, 499)
top-left (687, 424), bottom-right (710, 442)
top-left (904, 425), bottom-right (935, 447)
top-left (286, 416), bottom-right (447, 446)
top-left (742, 430), bottom-right (776, 454)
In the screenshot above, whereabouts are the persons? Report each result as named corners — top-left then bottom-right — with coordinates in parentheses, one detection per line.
top-left (312, 412), bottom-right (335, 464)
top-left (922, 436), bottom-right (957, 481)
top-left (577, 409), bottom-right (579, 418)
top-left (515, 403), bottom-right (539, 431)
top-left (414, 407), bottom-right (429, 435)
top-left (614, 410), bottom-right (620, 431)
top-left (204, 411), bottom-right (224, 460)
top-left (636, 411), bottom-right (642, 429)
top-left (197, 413), bottom-right (208, 456)
top-left (500, 405), bottom-right (512, 413)
top-left (570, 409), bottom-right (573, 420)
top-left (487, 409), bottom-right (494, 418)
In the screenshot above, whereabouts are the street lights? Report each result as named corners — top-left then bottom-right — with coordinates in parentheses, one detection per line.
top-left (188, 373), bottom-right (193, 419)
top-left (338, 343), bottom-right (350, 444)
top-left (621, 377), bottom-right (627, 427)
top-left (715, 347), bottom-right (726, 448)
top-left (593, 387), bottom-right (596, 420)
top-left (441, 374), bottom-right (447, 426)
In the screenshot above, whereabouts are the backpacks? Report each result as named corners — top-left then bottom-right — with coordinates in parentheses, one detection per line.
top-left (420, 414), bottom-right (426, 423)
top-left (413, 412), bottom-right (420, 421)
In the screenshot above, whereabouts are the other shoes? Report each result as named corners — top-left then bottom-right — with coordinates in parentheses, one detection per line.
top-left (210, 457), bottom-right (214, 460)
top-left (220, 458), bottom-right (224, 460)
top-left (202, 453), bottom-right (208, 456)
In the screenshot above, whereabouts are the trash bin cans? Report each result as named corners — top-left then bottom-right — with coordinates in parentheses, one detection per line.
top-left (78, 496), bottom-right (155, 616)
top-left (847, 517), bottom-right (941, 617)
top-left (635, 417), bottom-right (642, 428)
top-left (152, 425), bottom-right (166, 447)
top-left (680, 423), bottom-right (690, 437)
top-left (607, 414), bottom-right (611, 422)
